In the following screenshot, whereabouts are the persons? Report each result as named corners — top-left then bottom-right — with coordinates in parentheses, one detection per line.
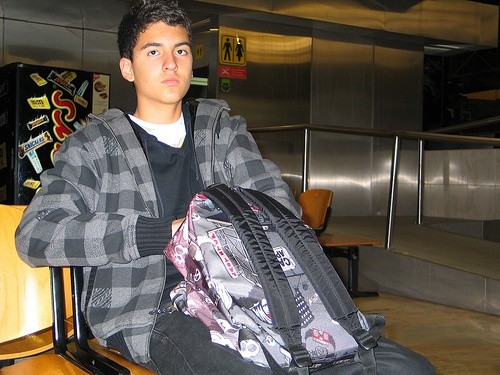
top-left (14, 0), bottom-right (439, 375)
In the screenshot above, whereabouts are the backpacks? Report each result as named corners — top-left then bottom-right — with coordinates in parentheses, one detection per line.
top-left (162, 181), bottom-right (385, 375)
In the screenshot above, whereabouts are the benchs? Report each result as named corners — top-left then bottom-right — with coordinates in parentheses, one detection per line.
top-left (314, 236), bottom-right (382, 299)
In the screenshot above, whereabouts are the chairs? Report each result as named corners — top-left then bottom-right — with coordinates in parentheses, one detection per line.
top-left (0, 203), bottom-right (160, 375)
top-left (297, 188), bottom-right (334, 235)
